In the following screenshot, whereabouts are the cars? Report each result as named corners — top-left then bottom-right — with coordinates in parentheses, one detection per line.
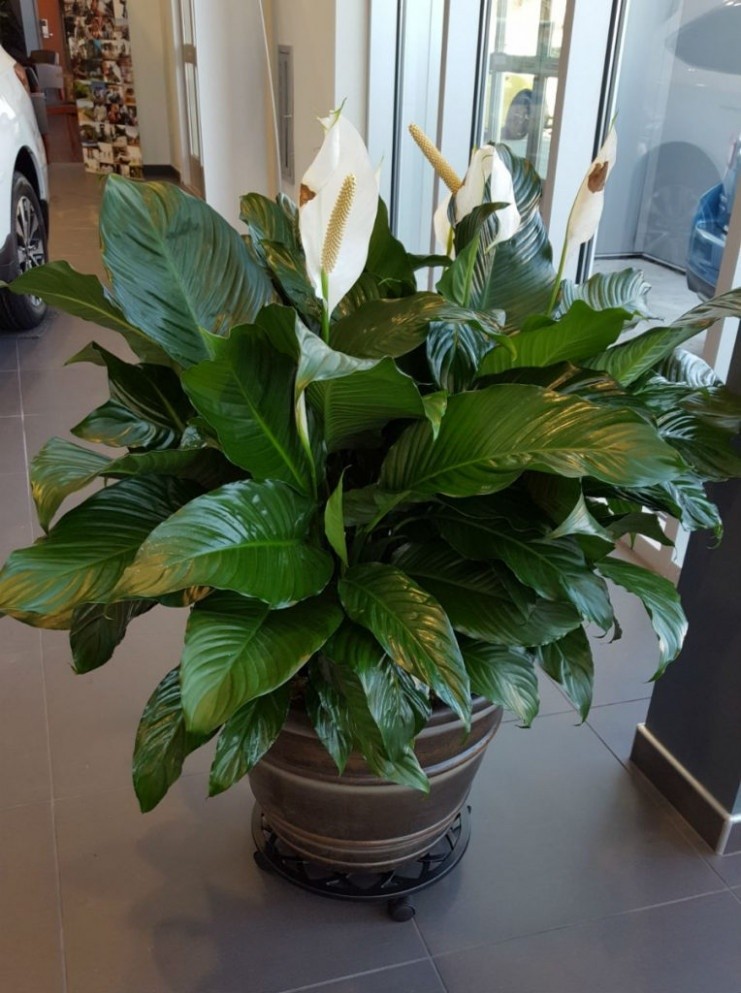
top-left (594, 3), bottom-right (740, 272)
top-left (0, 41), bottom-right (50, 330)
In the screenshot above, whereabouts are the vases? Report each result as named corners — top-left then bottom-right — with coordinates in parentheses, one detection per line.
top-left (244, 689), bottom-right (504, 876)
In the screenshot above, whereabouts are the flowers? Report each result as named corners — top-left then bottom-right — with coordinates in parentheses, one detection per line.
top-left (2, 92), bottom-right (741, 817)
